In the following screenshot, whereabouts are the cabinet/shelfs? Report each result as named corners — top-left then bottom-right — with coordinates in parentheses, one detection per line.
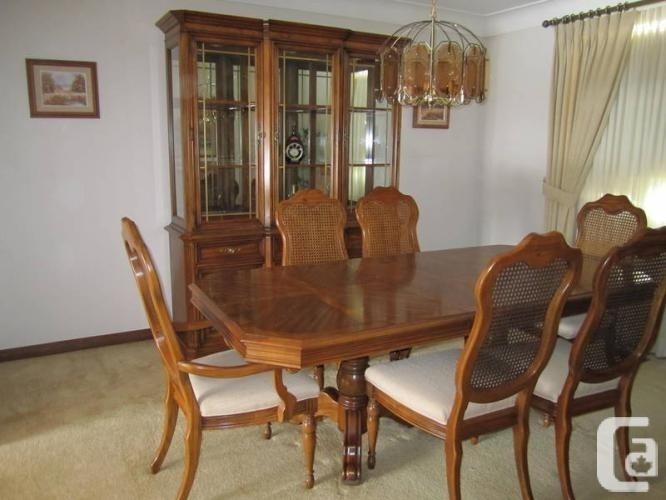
top-left (155, 7), bottom-right (413, 360)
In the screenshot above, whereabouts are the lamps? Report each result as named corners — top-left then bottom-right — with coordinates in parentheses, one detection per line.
top-left (376, 1), bottom-right (490, 110)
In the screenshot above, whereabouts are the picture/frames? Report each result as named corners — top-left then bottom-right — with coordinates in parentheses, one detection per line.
top-left (24, 57), bottom-right (101, 120)
top-left (412, 101), bottom-right (450, 128)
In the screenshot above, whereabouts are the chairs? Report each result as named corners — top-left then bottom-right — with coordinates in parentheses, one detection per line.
top-left (120, 181), bottom-right (665, 500)
